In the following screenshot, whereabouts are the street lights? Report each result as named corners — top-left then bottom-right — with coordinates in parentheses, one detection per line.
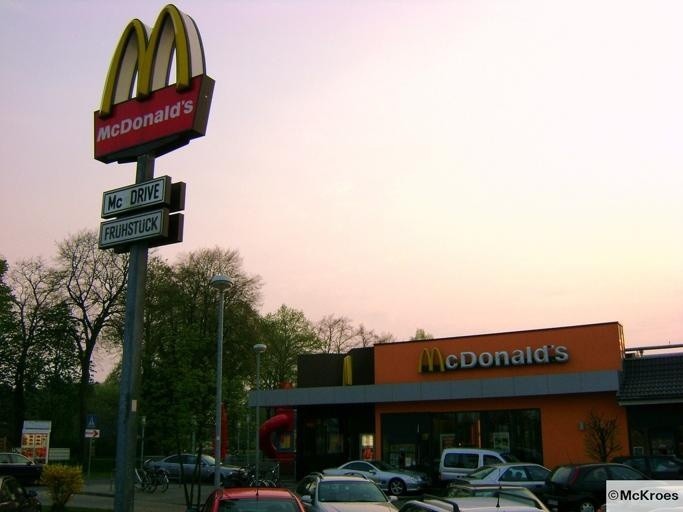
top-left (252, 343), bottom-right (267, 482)
top-left (208, 278), bottom-right (235, 486)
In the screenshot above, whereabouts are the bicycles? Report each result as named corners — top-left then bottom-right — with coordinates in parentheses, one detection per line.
top-left (222, 462), bottom-right (287, 487)
top-left (134, 458), bottom-right (170, 494)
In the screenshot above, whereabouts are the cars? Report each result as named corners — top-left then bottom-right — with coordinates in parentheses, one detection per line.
top-left (0, 451), bottom-right (49, 487)
top-left (300, 469), bottom-right (404, 512)
top-left (399, 462), bottom-right (649, 512)
top-left (143, 452), bottom-right (246, 484)
top-left (322, 458), bottom-right (434, 497)
top-left (202, 485), bottom-right (305, 512)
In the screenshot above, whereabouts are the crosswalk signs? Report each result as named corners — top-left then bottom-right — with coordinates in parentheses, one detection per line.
top-left (86, 415), bottom-right (97, 429)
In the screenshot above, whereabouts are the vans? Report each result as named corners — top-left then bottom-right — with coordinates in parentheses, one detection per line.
top-left (437, 447), bottom-right (509, 481)
